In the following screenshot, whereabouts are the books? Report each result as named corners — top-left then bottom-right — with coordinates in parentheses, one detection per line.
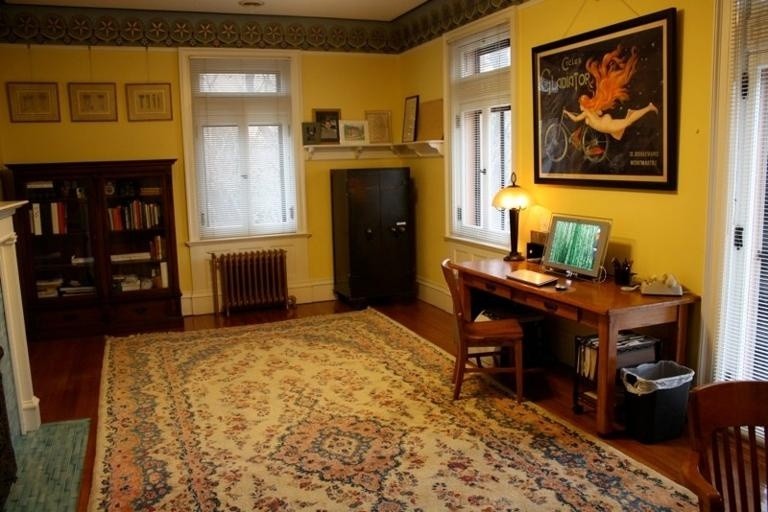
top-left (26, 180), bottom-right (168, 298)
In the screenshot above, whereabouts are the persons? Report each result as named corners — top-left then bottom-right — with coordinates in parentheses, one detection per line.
top-left (321, 120), bottom-right (337, 138)
top-left (563, 44), bottom-right (658, 141)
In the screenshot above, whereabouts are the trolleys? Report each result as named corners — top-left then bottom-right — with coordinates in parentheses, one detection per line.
top-left (569, 331), bottom-right (663, 439)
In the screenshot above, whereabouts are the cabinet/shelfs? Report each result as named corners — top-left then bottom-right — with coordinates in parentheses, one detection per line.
top-left (331, 167), bottom-right (417, 307)
top-left (2, 158), bottom-right (181, 337)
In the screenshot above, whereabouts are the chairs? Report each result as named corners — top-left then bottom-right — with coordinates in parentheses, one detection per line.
top-left (440, 257), bottom-right (525, 405)
top-left (681, 381), bottom-right (767, 510)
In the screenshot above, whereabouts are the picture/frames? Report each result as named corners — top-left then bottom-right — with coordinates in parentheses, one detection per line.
top-left (6, 80), bottom-right (61, 123)
top-left (531, 8), bottom-right (678, 191)
top-left (124, 82), bottom-right (172, 122)
top-left (311, 106), bottom-right (341, 142)
top-left (402, 96), bottom-right (420, 142)
top-left (301, 121), bottom-right (320, 145)
top-left (539, 210), bottom-right (612, 285)
top-left (68, 82), bottom-right (117, 123)
top-left (339, 119), bottom-right (370, 144)
top-left (365, 108), bottom-right (393, 143)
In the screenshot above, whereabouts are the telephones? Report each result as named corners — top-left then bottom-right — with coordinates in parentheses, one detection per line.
top-left (641, 272), bottom-right (683, 295)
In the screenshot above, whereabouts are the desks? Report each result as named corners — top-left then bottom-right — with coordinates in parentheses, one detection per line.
top-left (442, 241), bottom-right (697, 440)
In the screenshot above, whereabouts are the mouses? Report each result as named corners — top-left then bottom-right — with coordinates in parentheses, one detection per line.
top-left (554, 283), bottom-right (568, 290)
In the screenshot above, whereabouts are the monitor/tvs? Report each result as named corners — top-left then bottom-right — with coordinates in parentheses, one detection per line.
top-left (543, 211), bottom-right (613, 279)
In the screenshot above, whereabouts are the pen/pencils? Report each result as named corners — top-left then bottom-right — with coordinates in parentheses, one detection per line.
top-left (609, 255), bottom-right (633, 271)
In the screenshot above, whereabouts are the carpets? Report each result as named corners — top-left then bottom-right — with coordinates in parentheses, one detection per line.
top-left (2, 416), bottom-right (90, 512)
top-left (85, 304), bottom-right (701, 512)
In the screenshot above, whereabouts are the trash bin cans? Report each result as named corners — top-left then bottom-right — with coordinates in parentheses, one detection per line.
top-left (621, 360), bottom-right (695, 445)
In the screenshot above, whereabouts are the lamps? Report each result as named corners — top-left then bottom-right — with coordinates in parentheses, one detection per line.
top-left (493, 172), bottom-right (535, 261)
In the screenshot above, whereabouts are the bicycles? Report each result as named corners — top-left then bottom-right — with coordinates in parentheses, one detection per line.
top-left (544, 105), bottom-right (611, 164)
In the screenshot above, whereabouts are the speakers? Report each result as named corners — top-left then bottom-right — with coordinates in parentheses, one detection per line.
top-left (526, 242), bottom-right (544, 262)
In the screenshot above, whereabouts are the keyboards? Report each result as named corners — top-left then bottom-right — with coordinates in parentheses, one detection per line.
top-left (507, 268), bottom-right (559, 287)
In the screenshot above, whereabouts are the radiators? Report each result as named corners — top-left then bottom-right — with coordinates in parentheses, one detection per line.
top-left (206, 248), bottom-right (297, 317)
